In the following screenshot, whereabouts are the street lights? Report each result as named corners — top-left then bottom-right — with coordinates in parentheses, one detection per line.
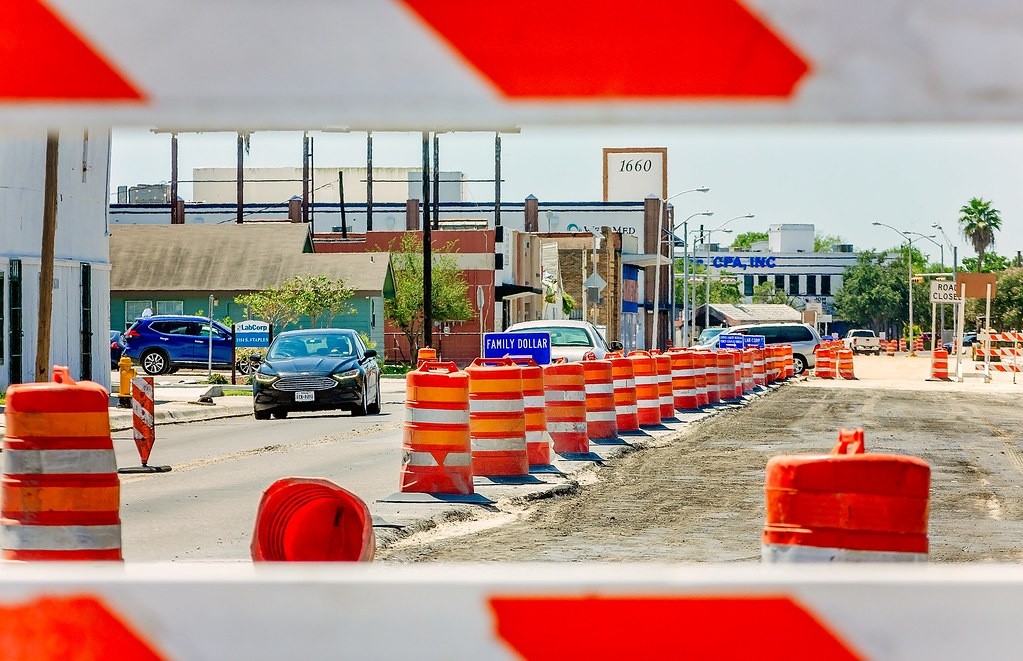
top-left (932, 223), bottom-right (956, 337)
top-left (901, 229), bottom-right (944, 349)
top-left (705, 228), bottom-right (734, 328)
top-left (667, 210), bottom-right (714, 340)
top-left (652, 185), bottom-right (709, 353)
top-left (871, 221), bottom-right (915, 355)
top-left (691, 213), bottom-right (756, 346)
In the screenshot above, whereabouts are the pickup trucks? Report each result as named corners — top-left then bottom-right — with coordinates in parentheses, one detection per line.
top-left (841, 328), bottom-right (882, 355)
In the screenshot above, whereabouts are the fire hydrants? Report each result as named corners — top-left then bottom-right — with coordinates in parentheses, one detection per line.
top-left (118, 355), bottom-right (139, 408)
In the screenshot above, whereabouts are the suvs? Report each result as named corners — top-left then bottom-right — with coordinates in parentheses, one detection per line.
top-left (124, 314), bottom-right (266, 378)
top-left (685, 322), bottom-right (823, 374)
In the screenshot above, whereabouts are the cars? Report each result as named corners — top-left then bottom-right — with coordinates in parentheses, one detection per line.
top-left (110, 329), bottom-right (125, 371)
top-left (905, 331), bottom-right (978, 355)
top-left (504, 320), bottom-right (623, 364)
top-left (693, 326), bottom-right (729, 350)
top-left (248, 329), bottom-right (383, 420)
